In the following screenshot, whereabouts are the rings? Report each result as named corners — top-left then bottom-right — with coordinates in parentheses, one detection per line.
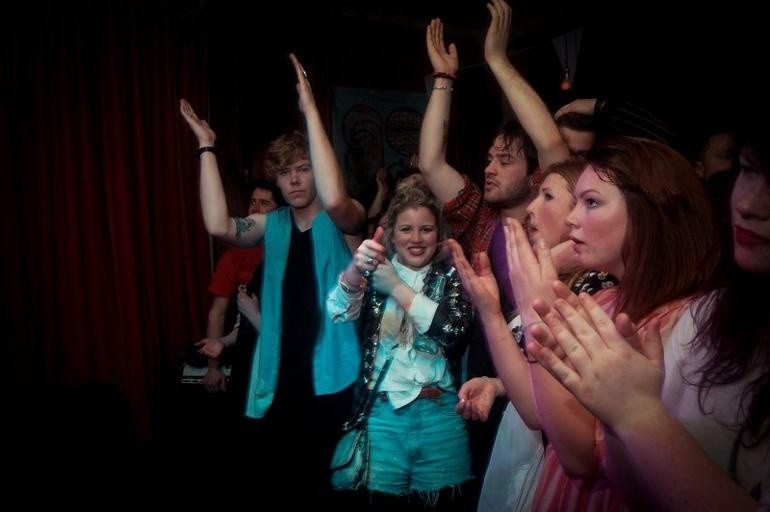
top-left (302, 70), bottom-right (309, 82)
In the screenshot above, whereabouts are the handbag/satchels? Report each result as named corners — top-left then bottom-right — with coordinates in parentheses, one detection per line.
top-left (329, 425), bottom-right (369, 492)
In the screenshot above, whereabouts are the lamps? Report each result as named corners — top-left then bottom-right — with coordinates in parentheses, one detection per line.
top-left (559, 18), bottom-right (573, 91)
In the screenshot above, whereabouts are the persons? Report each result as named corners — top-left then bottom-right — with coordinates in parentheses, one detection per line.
top-left (180, 50), bottom-right (366, 510)
top-left (202, 177), bottom-right (286, 396)
top-left (194, 254), bottom-right (262, 404)
top-left (327, 1), bottom-right (769, 508)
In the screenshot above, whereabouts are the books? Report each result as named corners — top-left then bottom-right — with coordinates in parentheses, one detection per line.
top-left (181, 362), bottom-right (234, 386)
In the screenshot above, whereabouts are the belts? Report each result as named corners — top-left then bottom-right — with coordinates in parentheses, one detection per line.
top-left (379, 388), bottom-right (442, 402)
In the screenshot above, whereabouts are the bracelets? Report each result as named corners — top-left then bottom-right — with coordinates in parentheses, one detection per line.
top-left (196, 146), bottom-right (219, 156)
